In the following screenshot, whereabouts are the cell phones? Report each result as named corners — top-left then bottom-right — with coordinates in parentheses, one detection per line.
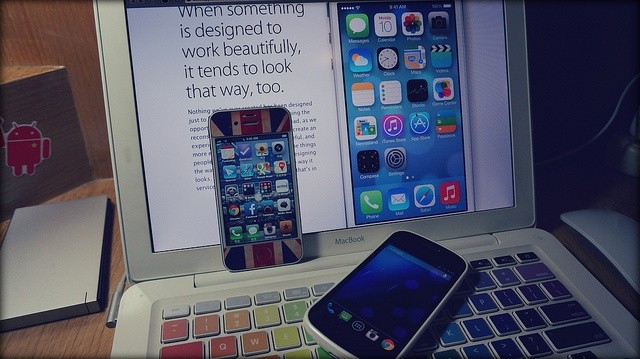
top-left (206, 106), bottom-right (305, 272)
top-left (303, 229), bottom-right (468, 359)
top-left (326, 0), bottom-right (475, 228)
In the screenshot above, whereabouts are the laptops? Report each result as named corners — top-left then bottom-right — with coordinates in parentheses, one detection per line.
top-left (91, 2), bottom-right (640, 358)
